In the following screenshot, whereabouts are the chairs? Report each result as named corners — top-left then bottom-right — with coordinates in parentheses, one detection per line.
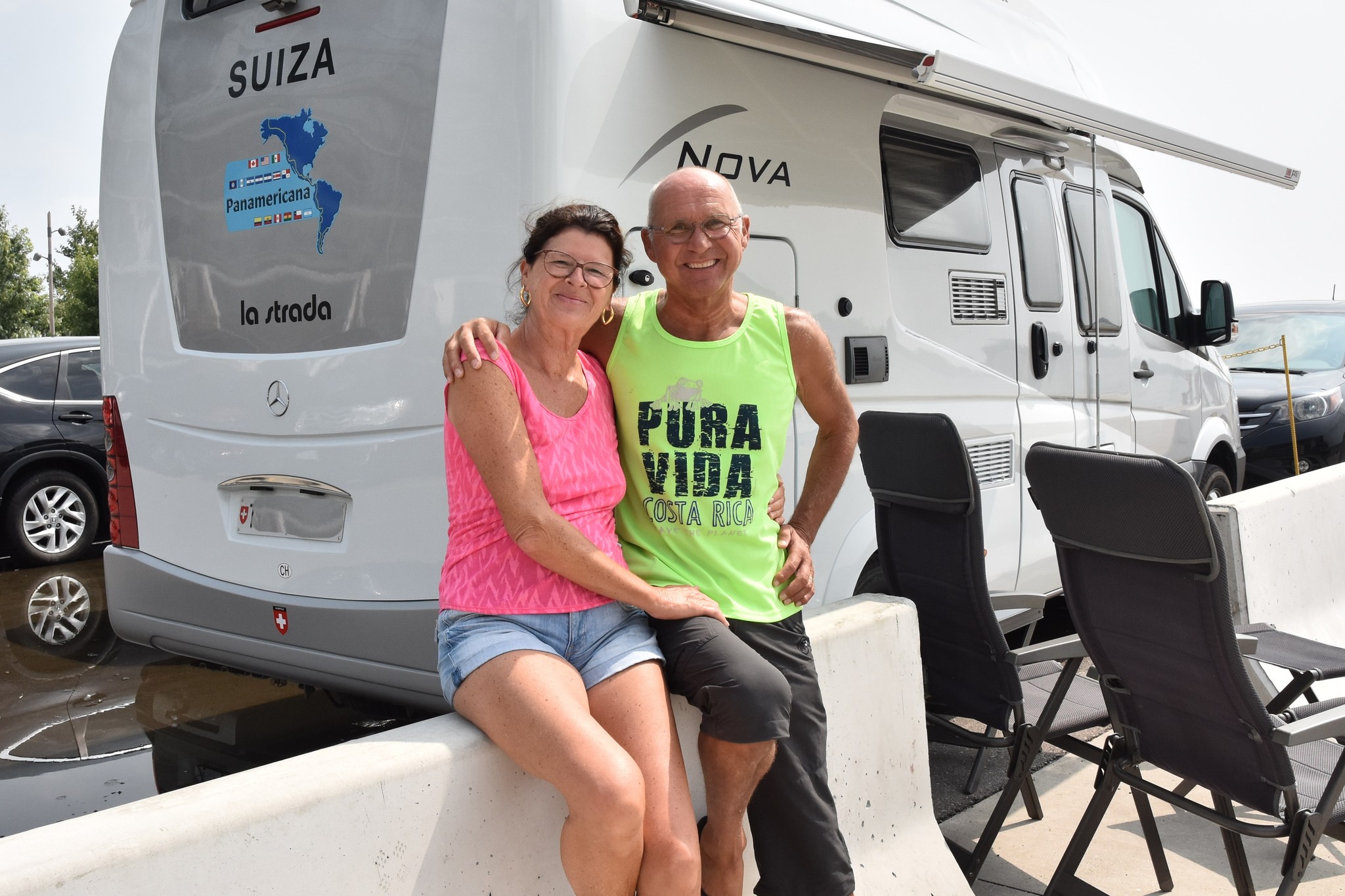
top-left (845, 406), bottom-right (1176, 895)
top-left (1232, 620), bottom-right (1344, 721)
top-left (1000, 442), bottom-right (1344, 895)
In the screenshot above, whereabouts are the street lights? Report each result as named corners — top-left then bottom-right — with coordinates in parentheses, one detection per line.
top-left (34, 207), bottom-right (66, 338)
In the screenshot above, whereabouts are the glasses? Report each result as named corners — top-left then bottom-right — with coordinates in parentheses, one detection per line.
top-left (533, 249), bottom-right (620, 289)
top-left (648, 213), bottom-right (741, 244)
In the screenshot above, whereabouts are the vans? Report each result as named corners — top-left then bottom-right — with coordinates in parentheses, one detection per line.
top-left (92, 0), bottom-right (1302, 736)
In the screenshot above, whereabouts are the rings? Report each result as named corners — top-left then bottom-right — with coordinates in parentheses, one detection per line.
top-left (808, 576), bottom-right (814, 582)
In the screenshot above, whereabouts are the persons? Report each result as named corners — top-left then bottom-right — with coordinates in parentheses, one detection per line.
top-left (433, 199), bottom-right (731, 896)
top-left (441, 165), bottom-right (864, 896)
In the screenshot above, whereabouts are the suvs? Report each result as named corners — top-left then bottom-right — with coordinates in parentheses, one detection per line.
top-left (1, 338), bottom-right (114, 567)
top-left (1209, 297), bottom-right (1344, 483)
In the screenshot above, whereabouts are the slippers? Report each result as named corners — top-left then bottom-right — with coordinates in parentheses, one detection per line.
top-left (696, 812), bottom-right (712, 896)
